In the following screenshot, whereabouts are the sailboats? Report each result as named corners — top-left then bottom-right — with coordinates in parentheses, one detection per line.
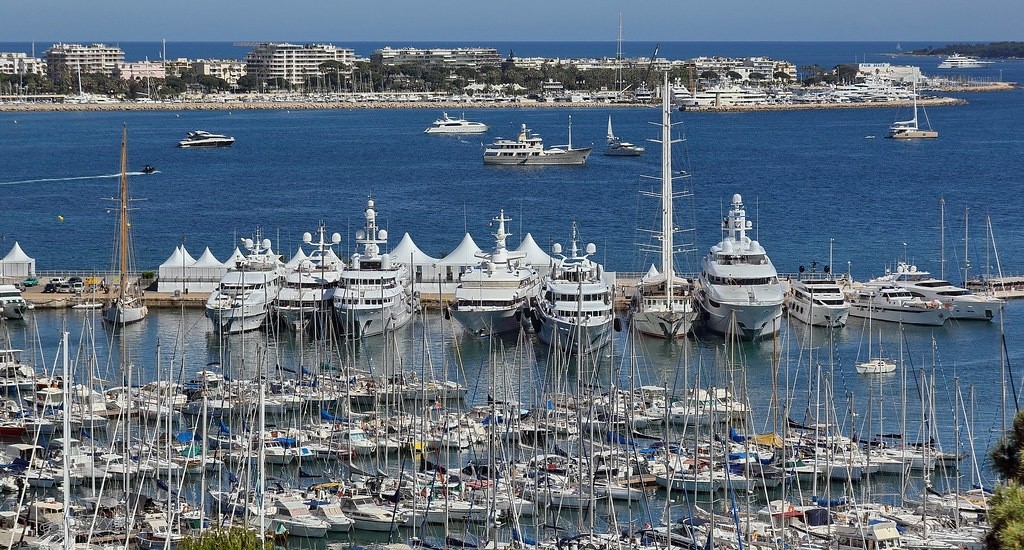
top-left (0, 71), bottom-right (1023, 550)
top-left (883, 67), bottom-right (940, 139)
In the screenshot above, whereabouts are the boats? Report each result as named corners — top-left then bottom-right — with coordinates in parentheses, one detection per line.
top-left (482, 115), bottom-right (593, 165)
top-left (178, 129), bottom-right (236, 148)
top-left (604, 115), bottom-right (646, 155)
top-left (937, 52), bottom-right (997, 70)
top-left (422, 112), bottom-right (490, 134)
